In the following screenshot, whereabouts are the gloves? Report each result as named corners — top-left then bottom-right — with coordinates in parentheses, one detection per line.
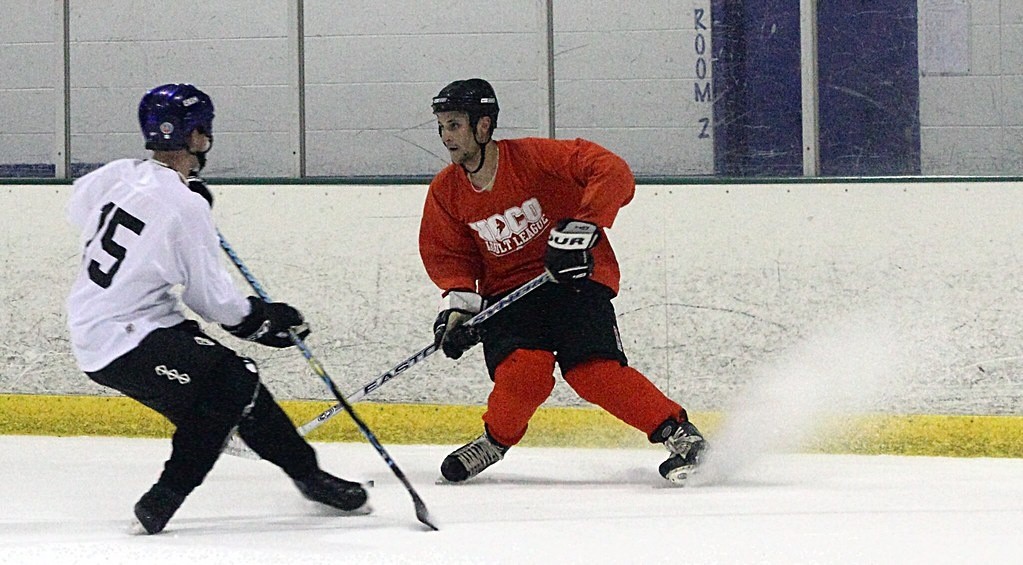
top-left (220, 295), bottom-right (312, 348)
top-left (434, 287), bottom-right (489, 360)
top-left (545, 218), bottom-right (601, 293)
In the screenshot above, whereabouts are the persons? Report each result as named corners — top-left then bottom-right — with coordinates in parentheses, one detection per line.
top-left (418, 78), bottom-right (718, 485)
top-left (65, 84), bottom-right (369, 536)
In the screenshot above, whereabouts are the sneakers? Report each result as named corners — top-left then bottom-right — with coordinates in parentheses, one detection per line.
top-left (649, 409), bottom-right (713, 486)
top-left (134, 484), bottom-right (179, 534)
top-left (439, 422), bottom-right (512, 482)
top-left (293, 468), bottom-right (368, 511)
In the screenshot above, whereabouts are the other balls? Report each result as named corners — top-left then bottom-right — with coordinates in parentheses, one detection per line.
top-left (361, 479), bottom-right (373, 489)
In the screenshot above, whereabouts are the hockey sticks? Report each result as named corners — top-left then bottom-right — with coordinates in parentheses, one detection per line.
top-left (220, 270), bottom-right (553, 461)
top-left (213, 226), bottom-right (437, 530)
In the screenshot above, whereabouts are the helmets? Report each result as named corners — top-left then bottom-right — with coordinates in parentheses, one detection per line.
top-left (431, 78), bottom-right (500, 146)
top-left (137, 83), bottom-right (215, 152)
